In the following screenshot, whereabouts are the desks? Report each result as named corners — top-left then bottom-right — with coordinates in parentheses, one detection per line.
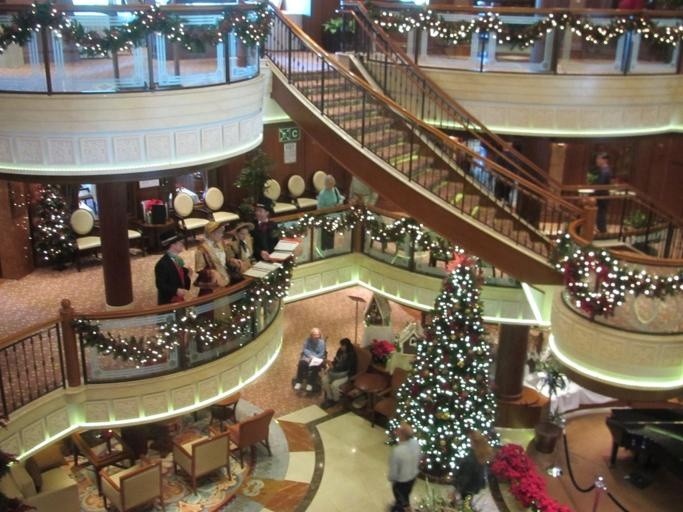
top-left (135, 217), bottom-right (177, 254)
top-left (524, 371), bottom-right (618, 415)
top-left (355, 373), bottom-right (387, 413)
top-left (72, 428), bottom-right (135, 495)
top-left (210, 392), bottom-right (240, 432)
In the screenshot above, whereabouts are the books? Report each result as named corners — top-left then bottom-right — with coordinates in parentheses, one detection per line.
top-left (269, 237), bottom-right (303, 262)
top-left (242, 260), bottom-right (282, 278)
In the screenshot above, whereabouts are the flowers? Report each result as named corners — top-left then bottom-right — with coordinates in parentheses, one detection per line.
top-left (371, 339), bottom-right (396, 359)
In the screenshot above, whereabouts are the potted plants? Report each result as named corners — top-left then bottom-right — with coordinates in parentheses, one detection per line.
top-left (323, 17), bottom-right (356, 52)
top-left (533, 353), bottom-right (565, 453)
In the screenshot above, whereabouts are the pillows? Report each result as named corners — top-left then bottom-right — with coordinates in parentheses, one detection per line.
top-left (32, 445), bottom-right (70, 473)
top-left (25, 457), bottom-right (42, 494)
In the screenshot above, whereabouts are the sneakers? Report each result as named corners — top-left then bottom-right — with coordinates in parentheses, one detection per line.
top-left (305, 383), bottom-right (311, 391)
top-left (293, 382), bottom-right (301, 389)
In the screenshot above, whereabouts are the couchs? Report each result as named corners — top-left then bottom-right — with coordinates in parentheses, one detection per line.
top-left (0, 461), bottom-right (80, 512)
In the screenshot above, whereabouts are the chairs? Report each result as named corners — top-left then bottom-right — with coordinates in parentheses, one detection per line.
top-left (372, 367), bottom-right (408, 427)
top-left (339, 347), bottom-right (372, 401)
top-left (174, 171), bottom-right (327, 250)
top-left (99, 410), bottom-right (275, 512)
top-left (71, 209), bottom-right (101, 272)
top-left (127, 226), bottom-right (145, 256)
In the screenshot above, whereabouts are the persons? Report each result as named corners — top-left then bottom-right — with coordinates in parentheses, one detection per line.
top-left (155, 230), bottom-right (193, 331)
top-left (387, 423), bottom-right (422, 512)
top-left (591, 152), bottom-right (612, 233)
top-left (250, 197), bottom-right (277, 261)
top-left (316, 174), bottom-right (342, 251)
top-left (193, 221), bottom-right (244, 323)
top-left (322, 339), bottom-right (357, 406)
top-left (455, 430), bottom-right (494, 500)
top-left (225, 221), bottom-right (255, 282)
top-left (294, 328), bottom-right (325, 392)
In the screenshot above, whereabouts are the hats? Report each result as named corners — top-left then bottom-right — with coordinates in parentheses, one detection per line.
top-left (205, 222), bottom-right (223, 236)
top-left (159, 228), bottom-right (183, 250)
top-left (227, 222), bottom-right (254, 237)
